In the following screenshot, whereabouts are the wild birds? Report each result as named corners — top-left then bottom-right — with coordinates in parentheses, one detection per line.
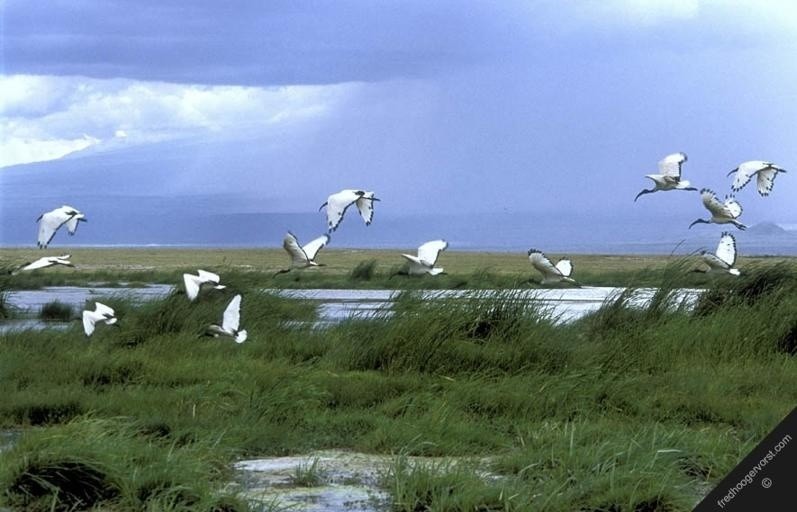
top-left (198, 294), bottom-right (250, 344)
top-left (166, 268), bottom-right (228, 300)
top-left (7, 253), bottom-right (75, 275)
top-left (684, 231), bottom-right (752, 286)
top-left (34, 204), bottom-right (87, 251)
top-left (82, 300), bottom-right (121, 338)
top-left (633, 146), bottom-right (787, 233)
top-left (387, 237), bottom-right (450, 281)
top-left (317, 187), bottom-right (383, 233)
top-left (518, 247), bottom-right (584, 290)
top-left (270, 230), bottom-right (330, 280)
